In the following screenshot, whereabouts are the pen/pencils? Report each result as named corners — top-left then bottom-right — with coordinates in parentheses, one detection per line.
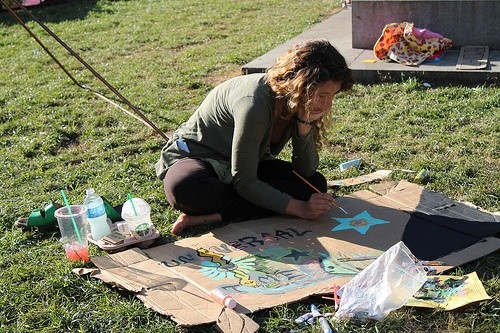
top-left (420, 261), bottom-right (446, 266)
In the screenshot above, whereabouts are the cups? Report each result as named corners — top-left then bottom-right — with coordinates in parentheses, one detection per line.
top-left (121, 198), bottom-right (152, 240)
top-left (53, 205), bottom-right (89, 260)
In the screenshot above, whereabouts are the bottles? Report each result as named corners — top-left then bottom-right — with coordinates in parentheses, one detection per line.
top-left (83, 187), bottom-right (112, 241)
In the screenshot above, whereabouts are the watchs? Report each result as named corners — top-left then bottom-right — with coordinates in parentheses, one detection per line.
top-left (296, 117), bottom-right (313, 127)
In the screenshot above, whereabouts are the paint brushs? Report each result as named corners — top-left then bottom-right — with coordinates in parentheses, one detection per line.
top-left (293, 171), bottom-right (350, 216)
top-left (337, 256), bottom-right (379, 261)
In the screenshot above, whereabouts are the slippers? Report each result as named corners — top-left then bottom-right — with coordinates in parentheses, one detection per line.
top-left (101, 196), bottom-right (125, 223)
top-left (14, 202), bottom-right (63, 227)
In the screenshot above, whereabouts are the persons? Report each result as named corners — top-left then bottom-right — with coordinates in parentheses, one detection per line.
top-left (155, 39), bottom-right (353, 235)
